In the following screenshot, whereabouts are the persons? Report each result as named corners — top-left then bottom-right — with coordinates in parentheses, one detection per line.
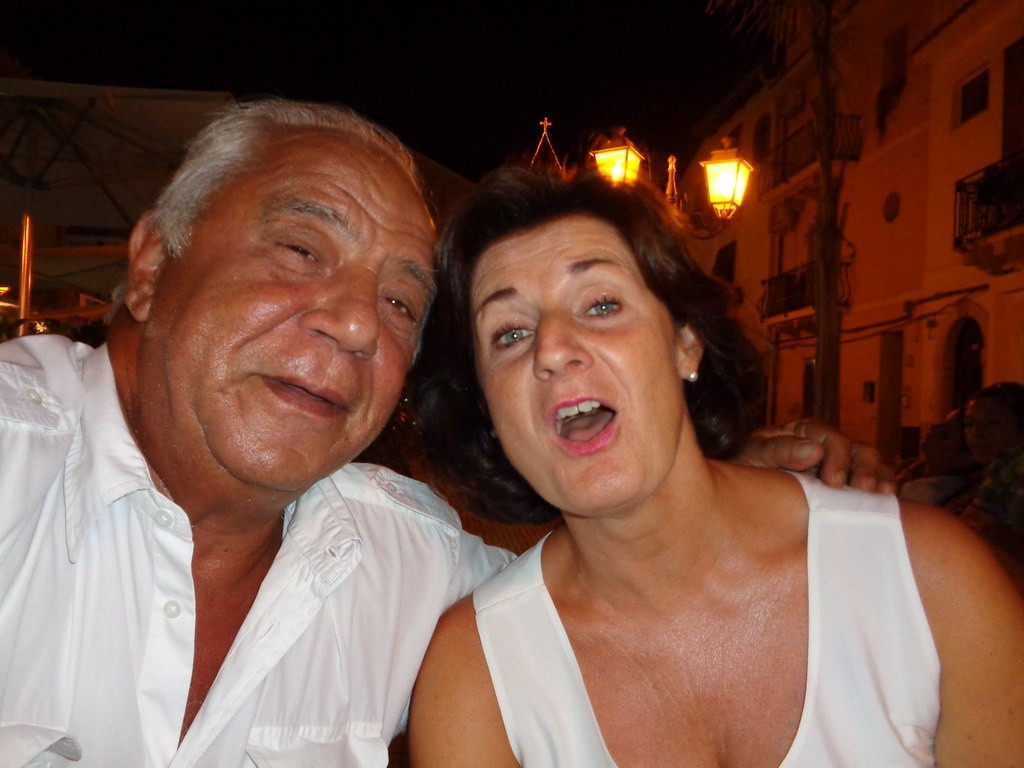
top-left (0, 102), bottom-right (902, 768)
top-left (392, 160), bottom-right (1024, 768)
